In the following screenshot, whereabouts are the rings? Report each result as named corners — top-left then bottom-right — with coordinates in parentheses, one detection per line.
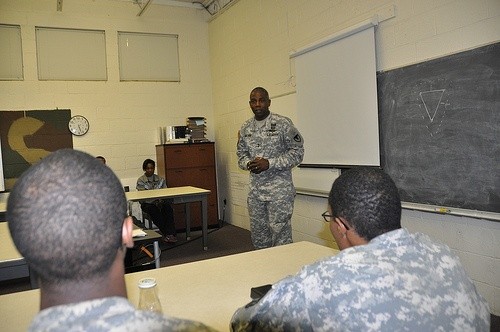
top-left (255, 166), bottom-right (258, 169)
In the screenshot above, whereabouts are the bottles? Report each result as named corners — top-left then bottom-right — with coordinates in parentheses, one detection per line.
top-left (136, 278), bottom-right (163, 316)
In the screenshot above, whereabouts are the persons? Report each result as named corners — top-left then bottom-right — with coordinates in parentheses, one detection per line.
top-left (96, 156), bottom-right (105, 164)
top-left (6, 148), bottom-right (218, 332)
top-left (231, 167), bottom-right (500, 332)
top-left (236, 87), bottom-right (304, 250)
top-left (135, 159), bottom-right (178, 243)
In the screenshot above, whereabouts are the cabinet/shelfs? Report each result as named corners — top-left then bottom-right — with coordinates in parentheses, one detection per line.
top-left (156, 142), bottom-right (219, 234)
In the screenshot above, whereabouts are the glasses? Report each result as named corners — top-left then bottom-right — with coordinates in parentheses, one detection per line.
top-left (322, 211), bottom-right (349, 230)
top-left (120, 201), bottom-right (132, 250)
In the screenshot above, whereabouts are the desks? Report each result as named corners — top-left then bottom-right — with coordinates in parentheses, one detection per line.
top-left (0, 220), bottom-right (162, 290)
top-left (0, 241), bottom-right (341, 332)
top-left (0, 185), bottom-right (211, 251)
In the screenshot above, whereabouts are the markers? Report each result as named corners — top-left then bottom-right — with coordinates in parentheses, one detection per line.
top-left (440, 208), bottom-right (451, 212)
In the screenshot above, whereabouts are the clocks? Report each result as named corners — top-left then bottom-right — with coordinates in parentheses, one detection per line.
top-left (67, 115), bottom-right (89, 136)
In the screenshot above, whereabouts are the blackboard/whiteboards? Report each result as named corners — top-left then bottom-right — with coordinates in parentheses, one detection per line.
top-left (268, 91), bottom-right (341, 198)
top-left (341, 40), bottom-right (500, 221)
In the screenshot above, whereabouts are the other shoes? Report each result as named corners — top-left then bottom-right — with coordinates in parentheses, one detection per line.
top-left (163, 235), bottom-right (178, 243)
